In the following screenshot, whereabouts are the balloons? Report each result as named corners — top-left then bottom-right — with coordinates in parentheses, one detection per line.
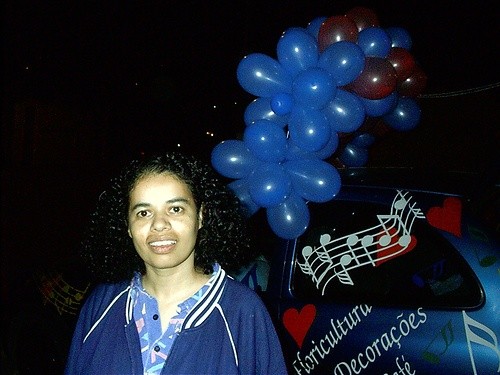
top-left (213, 6), bottom-right (428, 243)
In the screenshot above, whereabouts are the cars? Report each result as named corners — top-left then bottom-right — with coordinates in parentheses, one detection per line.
top-left (194, 166), bottom-right (500, 375)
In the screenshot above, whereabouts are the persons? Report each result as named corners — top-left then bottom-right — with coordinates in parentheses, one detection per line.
top-left (62, 151), bottom-right (293, 374)
